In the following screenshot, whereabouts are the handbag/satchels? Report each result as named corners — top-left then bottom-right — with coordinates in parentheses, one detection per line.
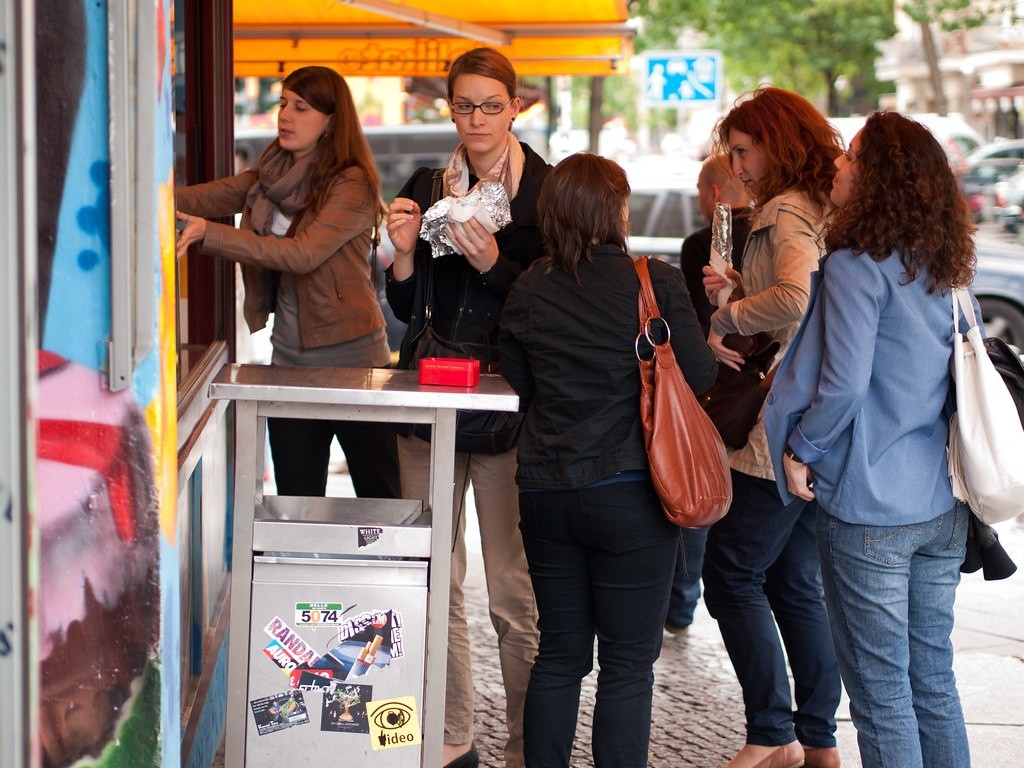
top-left (632, 255), bottom-right (734, 530)
top-left (699, 331), bottom-right (780, 450)
top-left (405, 319), bottom-right (526, 456)
top-left (945, 276), bottom-right (1024, 525)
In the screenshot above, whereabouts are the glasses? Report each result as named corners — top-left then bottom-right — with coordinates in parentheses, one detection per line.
top-left (451, 98), bottom-right (513, 114)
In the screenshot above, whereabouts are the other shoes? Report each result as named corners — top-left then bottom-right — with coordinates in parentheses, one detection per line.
top-left (799, 747), bottom-right (840, 768)
top-left (716, 738), bottom-right (806, 768)
top-left (442, 739), bottom-right (480, 767)
top-left (665, 623), bottom-right (689, 636)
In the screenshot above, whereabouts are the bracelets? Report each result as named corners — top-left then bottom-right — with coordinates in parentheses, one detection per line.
top-left (785, 447), bottom-right (803, 463)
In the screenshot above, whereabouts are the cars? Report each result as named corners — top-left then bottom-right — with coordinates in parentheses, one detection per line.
top-left (607, 110), bottom-right (1024, 434)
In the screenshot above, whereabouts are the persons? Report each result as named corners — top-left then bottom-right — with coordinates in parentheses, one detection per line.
top-left (385, 47), bottom-right (987, 768)
top-left (176, 66), bottom-right (402, 499)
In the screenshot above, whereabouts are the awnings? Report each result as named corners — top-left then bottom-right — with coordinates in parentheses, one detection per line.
top-left (234, 2), bottom-right (639, 76)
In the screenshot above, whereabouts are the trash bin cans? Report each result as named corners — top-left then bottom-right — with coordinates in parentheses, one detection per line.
top-left (207, 357), bottom-right (521, 767)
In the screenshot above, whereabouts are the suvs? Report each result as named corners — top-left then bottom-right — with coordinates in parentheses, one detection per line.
top-left (231, 120), bottom-right (550, 354)
